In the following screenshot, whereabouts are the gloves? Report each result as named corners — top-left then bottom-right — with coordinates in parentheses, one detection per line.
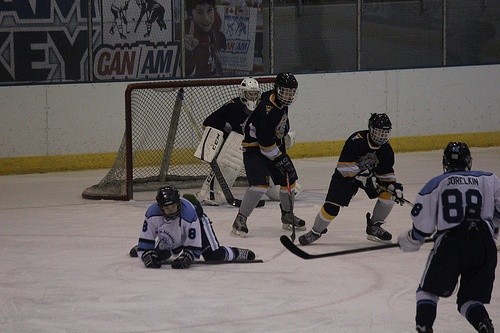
top-left (140, 248), bottom-right (161, 268)
top-left (171, 249), bottom-right (194, 269)
top-left (273, 151), bottom-right (297, 179)
top-left (384, 181), bottom-right (404, 206)
top-left (397, 229), bottom-right (426, 252)
top-left (355, 168), bottom-right (384, 199)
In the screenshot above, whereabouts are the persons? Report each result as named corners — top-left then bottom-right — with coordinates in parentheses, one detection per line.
top-left (130, 184), bottom-right (256, 270)
top-left (194, 76), bottom-right (303, 206)
top-left (183, 0), bottom-right (226, 76)
top-left (298, 112), bottom-right (405, 245)
top-left (397, 141), bottom-right (500, 333)
top-left (230, 72), bottom-right (307, 238)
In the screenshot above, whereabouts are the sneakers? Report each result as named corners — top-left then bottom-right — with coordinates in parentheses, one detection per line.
top-left (278, 203), bottom-right (306, 231)
top-left (366, 212), bottom-right (393, 243)
top-left (229, 213), bottom-right (248, 237)
top-left (298, 227), bottom-right (327, 245)
top-left (130, 245), bottom-right (139, 257)
top-left (221, 246), bottom-right (255, 262)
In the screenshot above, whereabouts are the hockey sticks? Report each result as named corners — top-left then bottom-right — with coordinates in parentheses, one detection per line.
top-left (279, 235), bottom-right (435, 260)
top-left (377, 183), bottom-right (414, 207)
top-left (180, 99), bottom-right (265, 208)
top-left (155, 246), bottom-right (286, 266)
top-left (282, 138), bottom-right (297, 242)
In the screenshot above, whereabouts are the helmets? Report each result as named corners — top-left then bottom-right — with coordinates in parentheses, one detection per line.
top-left (369, 112), bottom-right (392, 146)
top-left (240, 77), bottom-right (260, 111)
top-left (442, 141), bottom-right (472, 172)
top-left (274, 71), bottom-right (298, 106)
top-left (156, 184), bottom-right (181, 220)
